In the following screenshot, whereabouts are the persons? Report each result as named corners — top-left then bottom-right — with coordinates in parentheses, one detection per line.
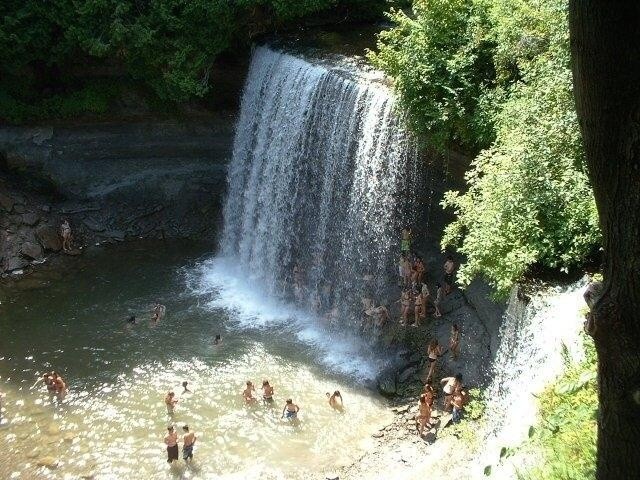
top-left (125, 305), bottom-right (163, 329)
top-left (61, 219), bottom-right (72, 251)
top-left (243, 378), bottom-right (343, 419)
top-left (164, 392), bottom-right (196, 463)
top-left (282, 219), bottom-right (453, 327)
top-left (29, 371), bottom-right (65, 400)
top-left (214, 334), bottom-right (222, 346)
top-left (415, 326), bottom-right (467, 436)
